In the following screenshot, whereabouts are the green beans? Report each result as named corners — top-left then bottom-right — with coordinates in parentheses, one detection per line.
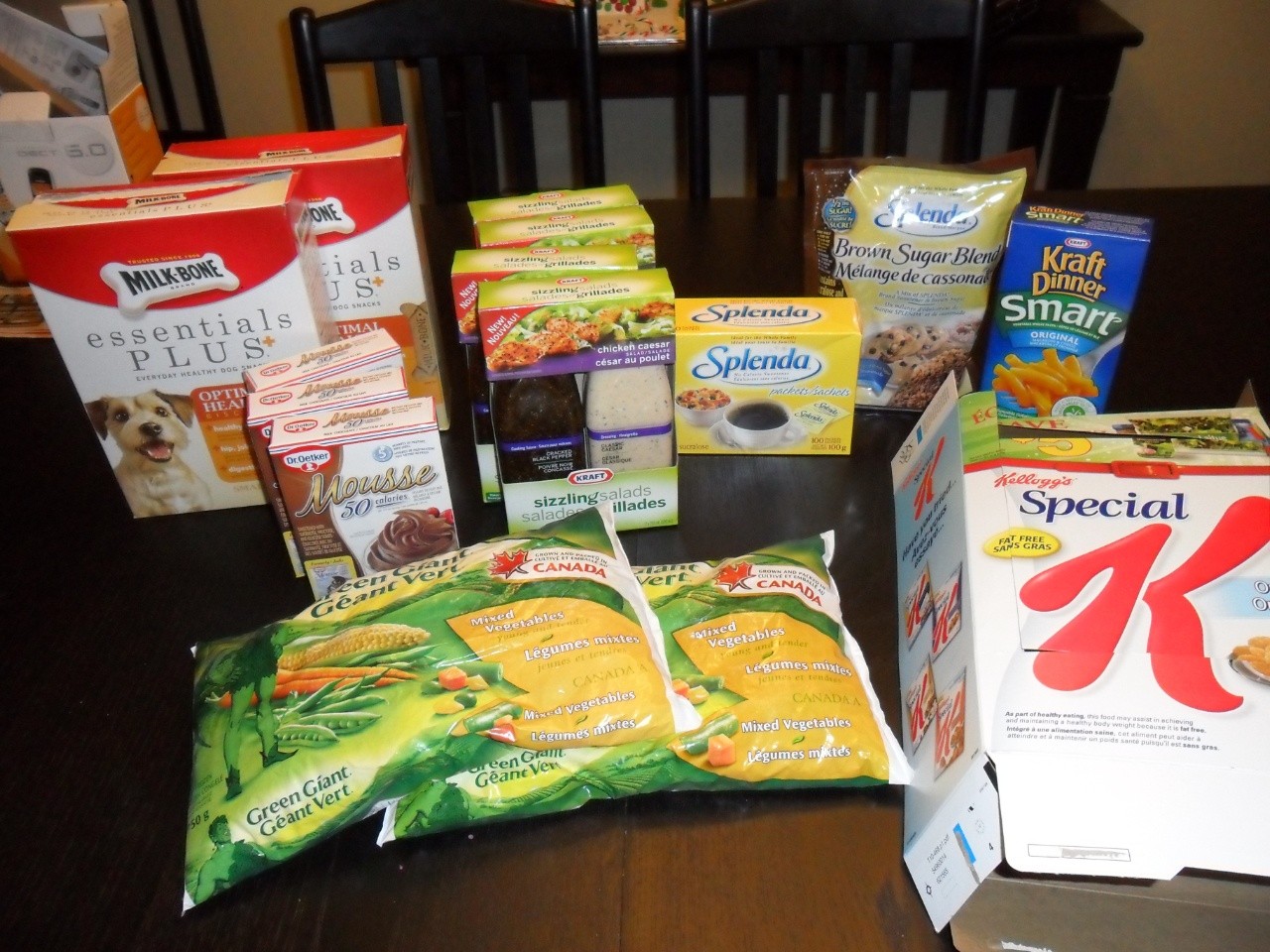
top-left (346, 646), bottom-right (444, 671)
top-left (272, 669), bottom-right (383, 746)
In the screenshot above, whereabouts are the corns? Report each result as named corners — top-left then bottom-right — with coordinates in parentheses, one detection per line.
top-left (276, 624), bottom-right (432, 670)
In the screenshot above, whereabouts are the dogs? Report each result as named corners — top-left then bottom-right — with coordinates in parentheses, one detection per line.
top-left (84, 388), bottom-right (211, 519)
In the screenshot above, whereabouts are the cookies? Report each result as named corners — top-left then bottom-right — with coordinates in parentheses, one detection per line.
top-left (864, 323), bottom-right (949, 364)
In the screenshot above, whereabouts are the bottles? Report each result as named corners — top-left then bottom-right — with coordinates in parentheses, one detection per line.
top-left (471, 347), bottom-right (674, 483)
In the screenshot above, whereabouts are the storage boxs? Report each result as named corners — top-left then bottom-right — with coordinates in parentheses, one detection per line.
top-left (982, 205), bottom-right (1152, 420)
top-left (0, 0), bottom-right (453, 523)
top-left (452, 190), bottom-right (863, 539)
top-left (880, 370), bottom-right (1270, 952)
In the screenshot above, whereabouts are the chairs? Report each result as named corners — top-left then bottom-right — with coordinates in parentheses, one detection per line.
top-left (289, 0), bottom-right (605, 204)
top-left (683, 0), bottom-right (999, 199)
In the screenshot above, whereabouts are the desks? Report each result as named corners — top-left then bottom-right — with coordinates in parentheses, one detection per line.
top-left (403, 0), bottom-right (1143, 205)
top-left (0, 180), bottom-right (1270, 952)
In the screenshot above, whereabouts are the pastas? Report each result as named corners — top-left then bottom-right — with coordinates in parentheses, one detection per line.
top-left (991, 349), bottom-right (1098, 417)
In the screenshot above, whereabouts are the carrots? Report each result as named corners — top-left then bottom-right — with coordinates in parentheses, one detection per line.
top-left (215, 666), bottom-right (416, 707)
